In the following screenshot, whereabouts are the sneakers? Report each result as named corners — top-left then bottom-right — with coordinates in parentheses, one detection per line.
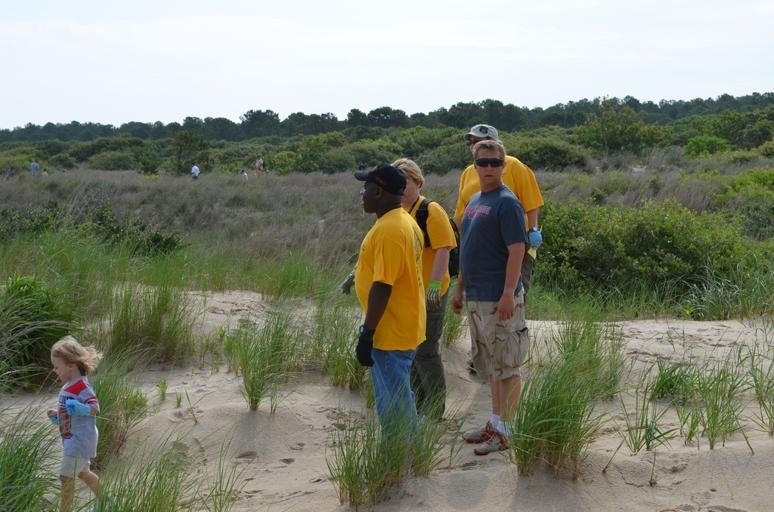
top-left (89, 498), bottom-right (116, 512)
top-left (462, 421), bottom-right (495, 443)
top-left (474, 428), bottom-right (510, 455)
top-left (417, 414), bottom-right (439, 427)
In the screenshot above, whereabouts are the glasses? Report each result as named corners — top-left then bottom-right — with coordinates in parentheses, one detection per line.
top-left (475, 158), bottom-right (504, 166)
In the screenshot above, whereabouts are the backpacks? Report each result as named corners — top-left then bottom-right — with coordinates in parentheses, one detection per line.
top-left (416, 198), bottom-right (460, 277)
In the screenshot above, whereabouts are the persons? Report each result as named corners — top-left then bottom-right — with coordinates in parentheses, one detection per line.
top-left (190, 162), bottom-right (201, 178)
top-left (353, 163), bottom-right (427, 474)
top-left (341, 158), bottom-right (457, 426)
top-left (47, 335), bottom-right (107, 511)
top-left (240, 154), bottom-right (264, 181)
top-left (451, 139), bottom-right (531, 455)
top-left (452, 123), bottom-right (544, 372)
top-left (31, 159), bottom-right (39, 176)
top-left (42, 168), bottom-right (49, 176)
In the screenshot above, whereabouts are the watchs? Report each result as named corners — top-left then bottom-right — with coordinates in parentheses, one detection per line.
top-left (359, 325), bottom-right (375, 336)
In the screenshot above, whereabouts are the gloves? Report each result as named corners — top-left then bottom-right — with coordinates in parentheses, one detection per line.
top-left (356, 330), bottom-right (374, 366)
top-left (66, 399), bottom-right (90, 416)
top-left (425, 280), bottom-right (441, 312)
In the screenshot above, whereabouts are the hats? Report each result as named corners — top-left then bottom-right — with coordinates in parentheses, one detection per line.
top-left (465, 124), bottom-right (498, 141)
top-left (354, 163), bottom-right (406, 195)
